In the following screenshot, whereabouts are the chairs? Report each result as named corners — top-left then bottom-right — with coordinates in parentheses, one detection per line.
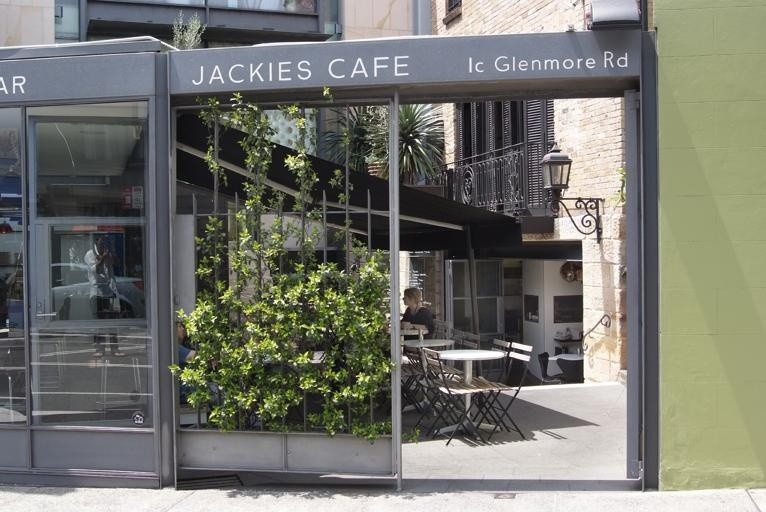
top-left (537, 347), bottom-right (584, 385)
top-left (400, 326), bottom-right (534, 448)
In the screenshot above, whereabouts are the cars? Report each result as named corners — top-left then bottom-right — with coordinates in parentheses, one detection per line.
top-left (4, 262), bottom-right (144, 339)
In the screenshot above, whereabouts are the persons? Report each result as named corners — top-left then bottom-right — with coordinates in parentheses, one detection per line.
top-left (84, 235), bottom-right (126, 358)
top-left (399, 287), bottom-right (437, 355)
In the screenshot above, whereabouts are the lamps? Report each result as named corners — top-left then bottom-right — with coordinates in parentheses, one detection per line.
top-left (538, 143), bottom-right (605, 244)
